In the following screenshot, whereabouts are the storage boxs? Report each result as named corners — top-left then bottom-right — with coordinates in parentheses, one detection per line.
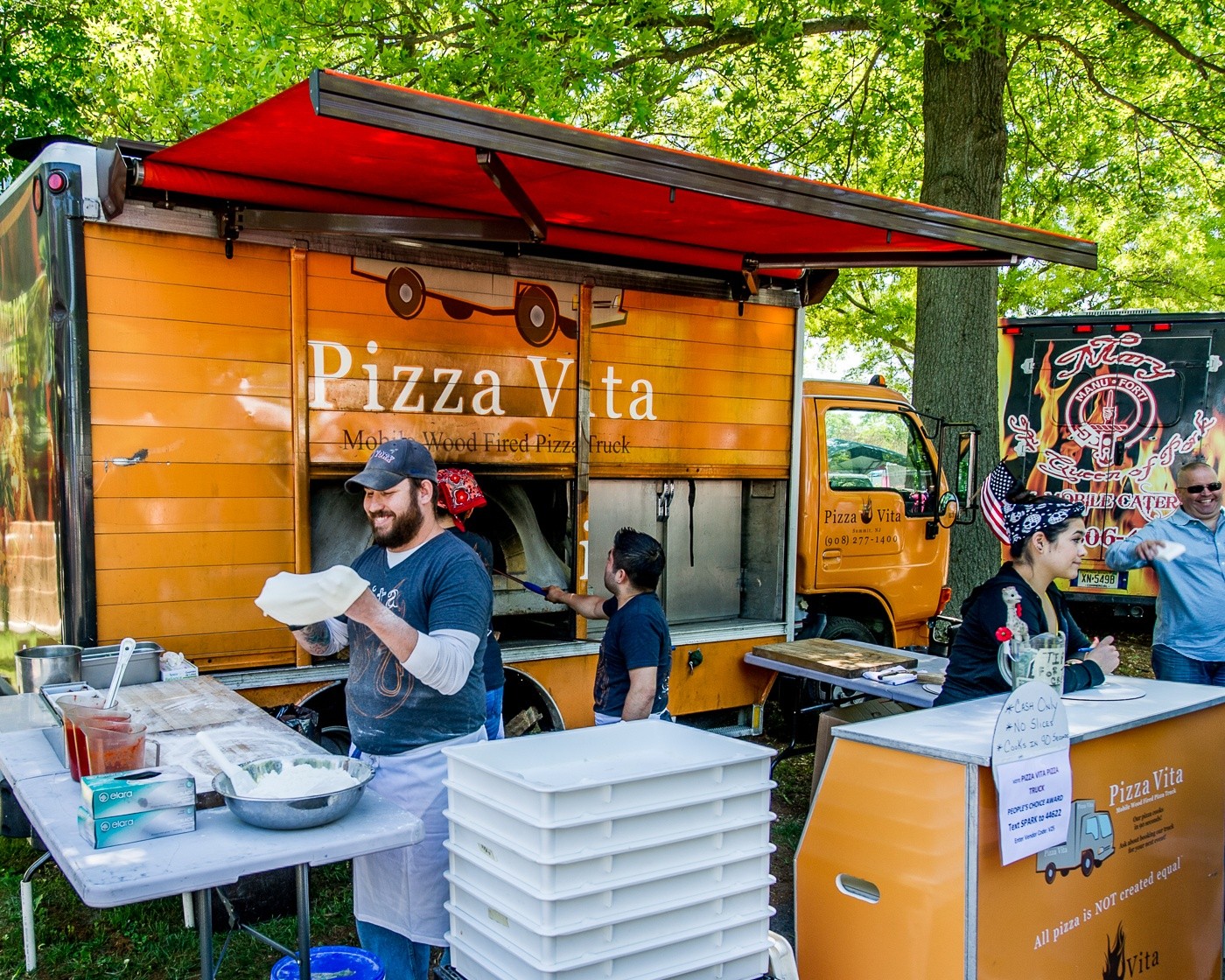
top-left (439, 718), bottom-right (779, 980)
top-left (80, 640), bottom-right (166, 689)
top-left (809, 695), bottom-right (928, 802)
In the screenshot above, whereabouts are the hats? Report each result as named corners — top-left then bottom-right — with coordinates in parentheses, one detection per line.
top-left (346, 437), bottom-right (437, 495)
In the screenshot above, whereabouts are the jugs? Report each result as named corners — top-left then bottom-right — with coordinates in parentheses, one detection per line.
top-left (77, 719), bottom-right (160, 776)
top-left (66, 706), bottom-right (132, 778)
top-left (56, 693), bottom-right (119, 781)
top-left (998, 633), bottom-right (1066, 699)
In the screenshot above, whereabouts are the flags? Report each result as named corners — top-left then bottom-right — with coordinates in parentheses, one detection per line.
top-left (979, 461), bottom-right (1018, 545)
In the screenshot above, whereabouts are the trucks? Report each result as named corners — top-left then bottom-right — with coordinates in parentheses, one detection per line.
top-left (0, 64), bottom-right (1099, 753)
top-left (994, 309), bottom-right (1224, 647)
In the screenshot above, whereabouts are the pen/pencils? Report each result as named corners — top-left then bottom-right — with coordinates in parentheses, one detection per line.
top-left (1078, 648), bottom-right (1094, 652)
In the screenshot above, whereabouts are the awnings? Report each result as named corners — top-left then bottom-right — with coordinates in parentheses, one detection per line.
top-left (134, 67), bottom-right (1098, 278)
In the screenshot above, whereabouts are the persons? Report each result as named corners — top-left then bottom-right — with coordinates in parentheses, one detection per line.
top-left (263, 438), bottom-right (505, 980)
top-left (1109, 462), bottom-right (1225, 686)
top-left (930, 484), bottom-right (1117, 707)
top-left (542, 527), bottom-right (675, 723)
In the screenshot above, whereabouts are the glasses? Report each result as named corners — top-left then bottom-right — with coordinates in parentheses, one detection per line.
top-left (1177, 482), bottom-right (1222, 493)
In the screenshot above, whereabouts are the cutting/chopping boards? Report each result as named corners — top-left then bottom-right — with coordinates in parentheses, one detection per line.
top-left (97, 674), bottom-right (332, 811)
top-left (753, 638), bottom-right (917, 679)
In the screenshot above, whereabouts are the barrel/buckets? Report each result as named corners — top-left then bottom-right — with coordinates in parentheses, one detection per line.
top-left (269, 945), bottom-right (386, 980)
top-left (14, 645), bottom-right (84, 694)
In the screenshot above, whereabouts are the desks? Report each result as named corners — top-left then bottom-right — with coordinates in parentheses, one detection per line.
top-left (0, 675), bottom-right (426, 980)
top-left (743, 640), bottom-right (951, 780)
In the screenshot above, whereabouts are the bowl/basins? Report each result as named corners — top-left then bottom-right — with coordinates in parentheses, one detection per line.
top-left (212, 753), bottom-right (376, 830)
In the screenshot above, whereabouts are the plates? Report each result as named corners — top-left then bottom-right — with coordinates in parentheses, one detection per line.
top-left (923, 684), bottom-right (942, 695)
